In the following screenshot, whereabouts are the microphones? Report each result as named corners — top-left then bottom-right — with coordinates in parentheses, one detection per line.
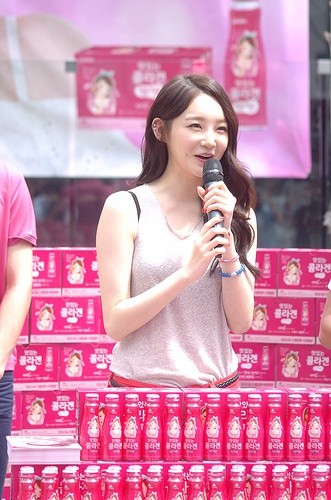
top-left (202, 159), bottom-right (224, 258)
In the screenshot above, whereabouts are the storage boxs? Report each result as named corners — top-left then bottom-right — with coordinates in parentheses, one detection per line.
top-left (76, 46), bottom-right (212, 130)
top-left (10, 248), bottom-right (331, 500)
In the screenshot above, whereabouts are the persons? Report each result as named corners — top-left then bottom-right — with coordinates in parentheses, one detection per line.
top-left (0, 137), bottom-right (38, 500)
top-left (96, 72), bottom-right (266, 388)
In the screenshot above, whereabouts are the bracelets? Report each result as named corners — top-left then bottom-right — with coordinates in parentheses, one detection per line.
top-left (217, 252), bottom-right (240, 263)
top-left (219, 263), bottom-right (245, 278)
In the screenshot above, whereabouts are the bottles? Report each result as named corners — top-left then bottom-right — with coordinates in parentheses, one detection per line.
top-left (16, 393), bottom-right (331, 500)
top-left (224, 0), bottom-right (268, 127)
